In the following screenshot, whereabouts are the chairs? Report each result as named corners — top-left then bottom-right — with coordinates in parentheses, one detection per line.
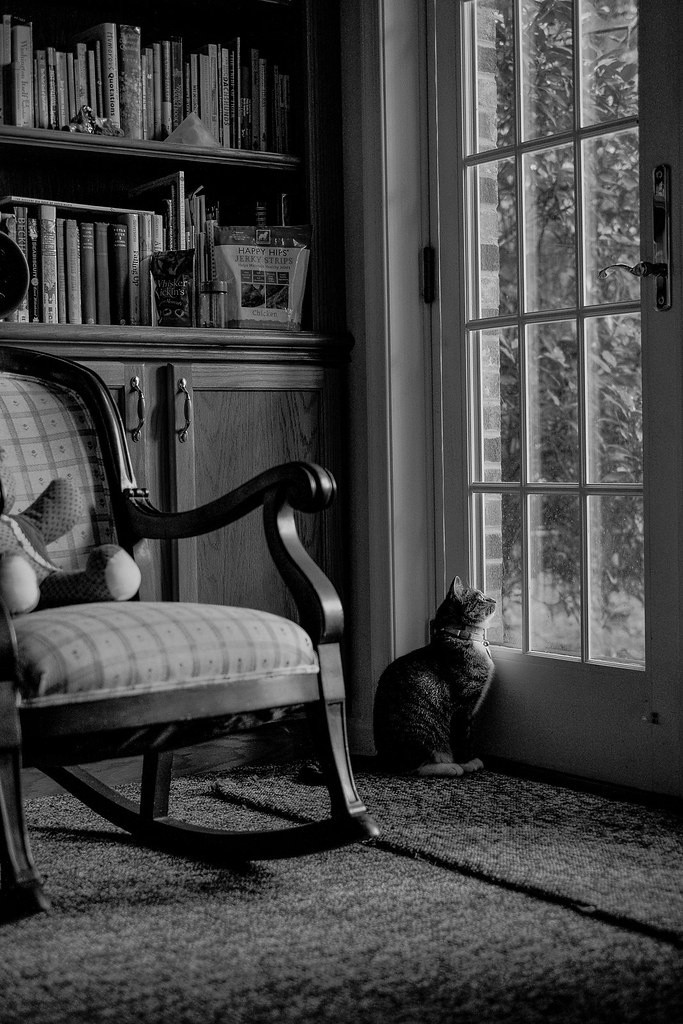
top-left (2, 345), bottom-right (379, 931)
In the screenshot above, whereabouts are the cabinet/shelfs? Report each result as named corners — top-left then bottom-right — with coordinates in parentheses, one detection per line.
top-left (1, 0), bottom-right (359, 799)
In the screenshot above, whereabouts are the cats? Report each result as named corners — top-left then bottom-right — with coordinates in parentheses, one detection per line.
top-left (302, 574), bottom-right (497, 785)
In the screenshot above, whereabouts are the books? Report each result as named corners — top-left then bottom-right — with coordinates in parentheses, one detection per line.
top-left (0, 14), bottom-right (294, 154)
top-left (0, 169), bottom-right (293, 328)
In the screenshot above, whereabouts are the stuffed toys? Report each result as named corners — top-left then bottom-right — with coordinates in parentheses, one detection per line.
top-left (0, 467), bottom-right (142, 615)
